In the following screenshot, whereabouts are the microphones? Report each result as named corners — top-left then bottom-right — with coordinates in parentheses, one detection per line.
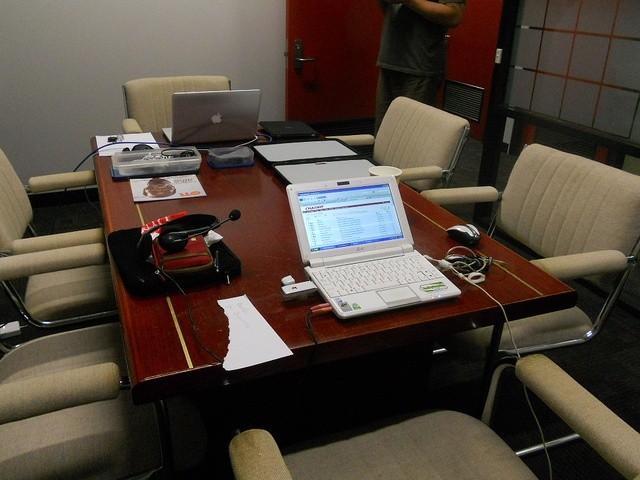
top-left (187, 209), bottom-right (241, 239)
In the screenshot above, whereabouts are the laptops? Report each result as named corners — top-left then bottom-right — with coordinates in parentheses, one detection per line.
top-left (161, 90), bottom-right (262, 145)
top-left (286, 176), bottom-right (461, 321)
top-left (259, 119), bottom-right (320, 138)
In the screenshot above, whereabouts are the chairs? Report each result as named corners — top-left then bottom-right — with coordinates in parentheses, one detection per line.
top-left (326, 95), bottom-right (470, 188)
top-left (1, 242), bottom-right (131, 480)
top-left (120, 74), bottom-right (232, 133)
top-left (420, 142), bottom-right (640, 395)
top-left (226, 353), bottom-right (640, 480)
top-left (0, 147), bottom-right (104, 252)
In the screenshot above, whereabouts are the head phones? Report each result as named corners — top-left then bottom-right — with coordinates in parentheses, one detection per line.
top-left (135, 212), bottom-right (219, 263)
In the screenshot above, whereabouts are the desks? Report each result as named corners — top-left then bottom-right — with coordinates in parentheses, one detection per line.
top-left (91, 120), bottom-right (576, 407)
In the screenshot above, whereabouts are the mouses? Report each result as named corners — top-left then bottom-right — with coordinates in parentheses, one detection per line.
top-left (447, 223), bottom-right (481, 245)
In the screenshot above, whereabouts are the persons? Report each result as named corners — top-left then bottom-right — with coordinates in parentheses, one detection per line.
top-left (374, 0), bottom-right (467, 138)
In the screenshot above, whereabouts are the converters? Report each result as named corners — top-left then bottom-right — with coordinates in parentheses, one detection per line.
top-left (1, 321), bottom-right (21, 339)
